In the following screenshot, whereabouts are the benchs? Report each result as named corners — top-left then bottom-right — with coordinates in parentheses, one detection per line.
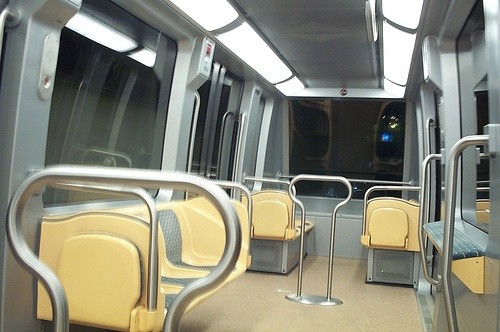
top-left (361, 196), bottom-right (421, 287)
top-left (36, 194), bottom-right (252, 332)
top-left (243, 189), bottom-right (315, 274)
